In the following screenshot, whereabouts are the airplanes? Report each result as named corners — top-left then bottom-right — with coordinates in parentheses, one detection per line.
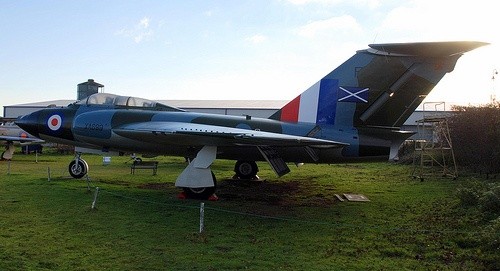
top-left (0, 42), bottom-right (491, 200)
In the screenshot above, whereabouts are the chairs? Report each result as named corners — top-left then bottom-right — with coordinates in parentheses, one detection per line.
top-left (102, 96), bottom-right (114, 105)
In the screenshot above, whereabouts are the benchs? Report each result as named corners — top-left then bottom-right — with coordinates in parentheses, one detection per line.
top-left (131, 161), bottom-right (159, 176)
